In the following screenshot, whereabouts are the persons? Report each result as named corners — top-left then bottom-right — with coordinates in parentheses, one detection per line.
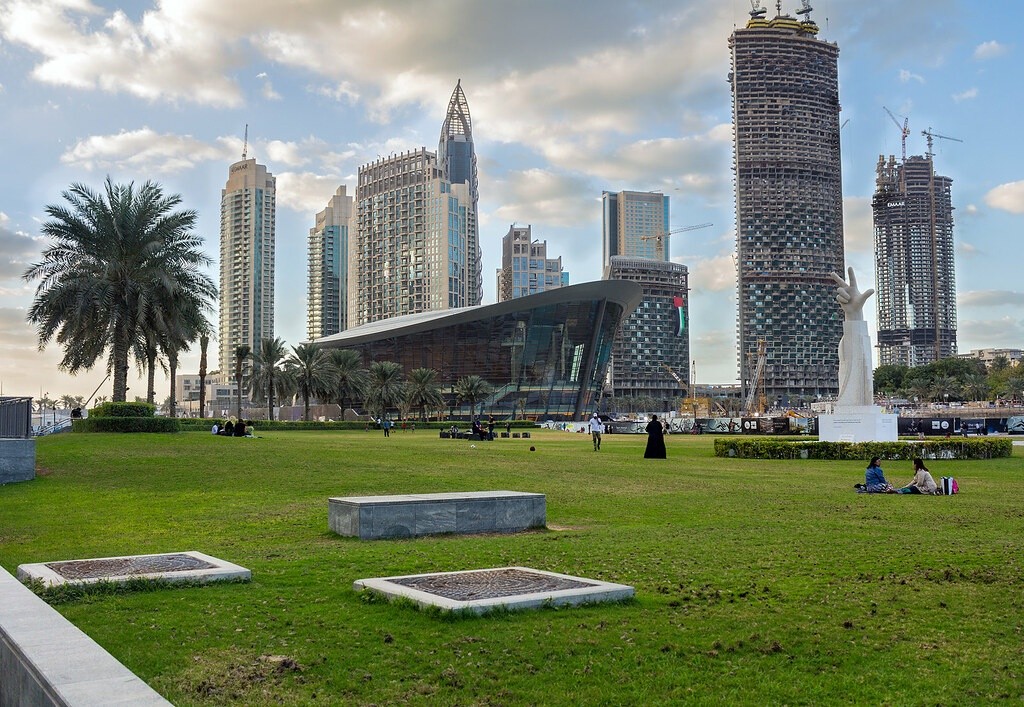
top-left (71, 408), bottom-right (83, 426)
top-left (890, 458), bottom-right (937, 495)
top-left (383, 417), bottom-right (415, 437)
top-left (376, 417), bottom-right (381, 429)
top-left (866, 456), bottom-right (893, 492)
top-left (894, 405), bottom-right (900, 417)
top-left (830, 266), bottom-right (874, 321)
top-left (692, 421), bottom-right (702, 434)
top-left (961, 421), bottom-right (968, 438)
top-left (440, 414), bottom-right (496, 441)
top-left (730, 421), bottom-right (735, 434)
top-left (589, 413), bottom-right (601, 452)
top-left (663, 418), bottom-right (670, 435)
top-left (212, 415), bottom-right (254, 437)
top-left (644, 415), bottom-right (667, 459)
top-left (995, 398), bottom-right (1000, 409)
top-left (982, 426), bottom-right (989, 435)
top-left (916, 418), bottom-right (924, 440)
top-left (976, 422), bottom-right (981, 435)
top-left (505, 422), bottom-right (510, 439)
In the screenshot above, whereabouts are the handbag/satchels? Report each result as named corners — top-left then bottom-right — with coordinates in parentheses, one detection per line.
top-left (940, 475), bottom-right (953, 495)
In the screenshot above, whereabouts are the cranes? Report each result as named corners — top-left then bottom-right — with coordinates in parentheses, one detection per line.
top-left (921, 126), bottom-right (964, 359)
top-left (883, 107), bottom-right (911, 191)
top-left (664, 360), bottom-right (696, 418)
top-left (640, 223), bottom-right (714, 260)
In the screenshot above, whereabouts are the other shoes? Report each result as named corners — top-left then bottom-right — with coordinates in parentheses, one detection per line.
top-left (598, 446), bottom-right (600, 450)
top-left (593, 448), bottom-right (596, 451)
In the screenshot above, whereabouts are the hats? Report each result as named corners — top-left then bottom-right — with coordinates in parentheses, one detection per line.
top-left (593, 413), bottom-right (597, 417)
